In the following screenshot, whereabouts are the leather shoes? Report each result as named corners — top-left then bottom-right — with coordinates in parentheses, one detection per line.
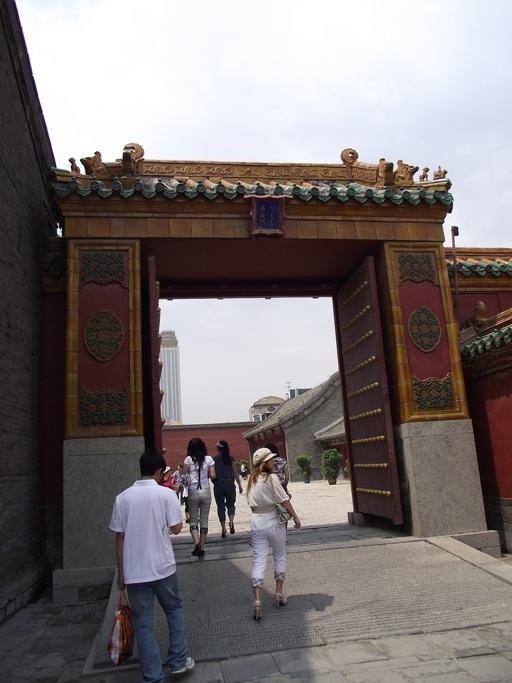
top-left (221, 528), bottom-right (227, 538)
top-left (192, 542), bottom-right (205, 557)
top-left (228, 523), bottom-right (235, 535)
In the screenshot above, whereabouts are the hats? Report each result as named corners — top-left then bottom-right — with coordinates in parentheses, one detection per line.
top-left (216, 440), bottom-right (224, 447)
top-left (163, 465), bottom-right (171, 473)
top-left (252, 447), bottom-right (278, 466)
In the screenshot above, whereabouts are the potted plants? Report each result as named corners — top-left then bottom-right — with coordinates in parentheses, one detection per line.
top-left (321, 449), bottom-right (342, 485)
top-left (296, 455), bottom-right (311, 483)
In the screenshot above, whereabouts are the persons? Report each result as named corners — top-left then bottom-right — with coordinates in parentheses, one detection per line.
top-left (241, 461), bottom-right (246, 480)
top-left (181, 436), bottom-right (216, 559)
top-left (245, 446), bottom-right (302, 620)
top-left (264, 442), bottom-right (290, 532)
top-left (208, 439), bottom-right (243, 540)
top-left (109, 449), bottom-right (195, 682)
top-left (164, 463), bottom-right (190, 524)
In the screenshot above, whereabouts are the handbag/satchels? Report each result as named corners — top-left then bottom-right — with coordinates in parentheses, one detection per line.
top-left (274, 503), bottom-right (292, 524)
top-left (108, 587), bottom-right (134, 666)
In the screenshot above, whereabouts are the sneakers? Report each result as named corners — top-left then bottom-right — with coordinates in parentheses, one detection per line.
top-left (171, 656), bottom-right (195, 674)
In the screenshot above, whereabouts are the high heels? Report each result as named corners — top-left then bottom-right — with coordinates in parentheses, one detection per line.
top-left (274, 592), bottom-right (287, 608)
top-left (254, 599), bottom-right (263, 619)
